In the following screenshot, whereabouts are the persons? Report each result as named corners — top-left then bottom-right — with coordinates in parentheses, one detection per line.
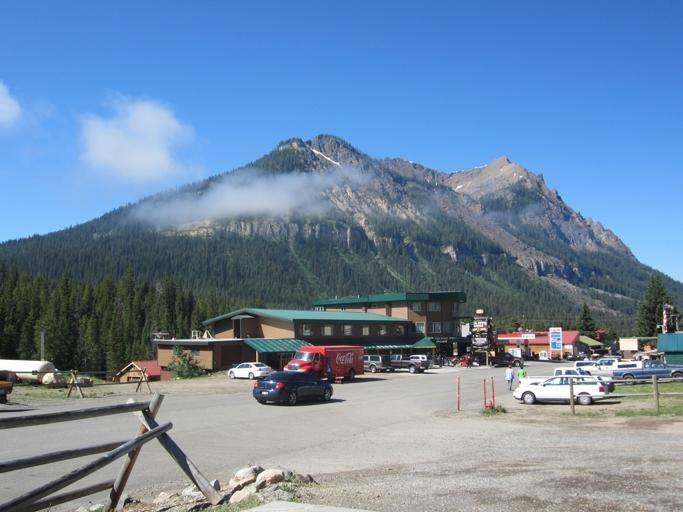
top-left (517, 365), bottom-right (526, 383)
top-left (466, 353), bottom-right (473, 368)
top-left (584, 356), bottom-right (589, 361)
top-left (505, 364), bottom-right (514, 391)
top-left (426, 351), bottom-right (448, 367)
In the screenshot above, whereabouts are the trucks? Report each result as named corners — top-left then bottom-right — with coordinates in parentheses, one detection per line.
top-left (283, 346), bottom-right (364, 380)
top-left (657, 333), bottom-right (683, 381)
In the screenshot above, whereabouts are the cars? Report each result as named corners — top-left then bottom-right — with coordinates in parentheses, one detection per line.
top-left (512, 366), bottom-right (615, 405)
top-left (227, 362), bottom-right (272, 380)
top-left (563, 352), bottom-right (595, 366)
top-left (253, 371), bottom-right (333, 405)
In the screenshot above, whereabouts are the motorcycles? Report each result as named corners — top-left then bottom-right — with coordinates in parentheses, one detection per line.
top-left (466, 355), bottom-right (473, 368)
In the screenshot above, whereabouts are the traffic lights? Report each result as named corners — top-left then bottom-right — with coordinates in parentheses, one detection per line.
top-left (524, 340), bottom-right (528, 350)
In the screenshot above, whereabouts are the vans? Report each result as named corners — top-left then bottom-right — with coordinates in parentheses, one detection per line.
top-left (409, 355), bottom-right (433, 369)
top-left (488, 353), bottom-right (522, 367)
top-left (364, 355), bottom-right (390, 373)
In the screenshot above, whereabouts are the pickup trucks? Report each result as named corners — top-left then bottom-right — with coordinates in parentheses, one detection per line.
top-left (591, 359), bottom-right (683, 385)
top-left (390, 354), bottom-right (430, 373)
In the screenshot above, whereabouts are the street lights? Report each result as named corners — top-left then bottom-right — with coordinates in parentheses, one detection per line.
top-left (657, 325), bottom-right (665, 334)
